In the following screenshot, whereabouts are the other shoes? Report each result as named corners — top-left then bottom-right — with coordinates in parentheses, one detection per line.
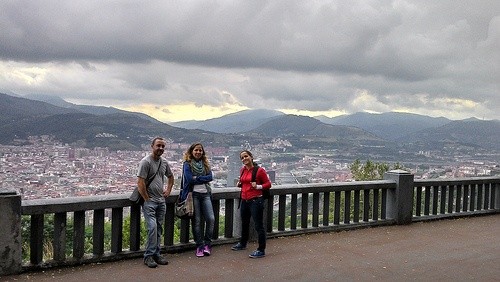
top-left (143, 255), bottom-right (158, 268)
top-left (152, 254), bottom-right (168, 265)
top-left (248, 248), bottom-right (266, 258)
top-left (231, 242), bottom-right (246, 251)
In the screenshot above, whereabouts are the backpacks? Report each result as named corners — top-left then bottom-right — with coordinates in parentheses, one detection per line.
top-left (240, 166), bottom-right (275, 200)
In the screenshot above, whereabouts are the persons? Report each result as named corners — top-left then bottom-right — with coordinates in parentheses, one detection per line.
top-left (136, 137), bottom-right (174, 267)
top-left (182, 143), bottom-right (215, 257)
top-left (231, 150), bottom-right (271, 258)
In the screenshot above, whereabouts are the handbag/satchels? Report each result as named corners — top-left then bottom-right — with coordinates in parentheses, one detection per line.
top-left (176, 191), bottom-right (194, 220)
top-left (128, 187), bottom-right (144, 211)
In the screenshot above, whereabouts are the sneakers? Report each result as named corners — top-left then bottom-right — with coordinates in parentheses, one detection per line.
top-left (194, 245), bottom-right (205, 257)
top-left (203, 242), bottom-right (212, 256)
